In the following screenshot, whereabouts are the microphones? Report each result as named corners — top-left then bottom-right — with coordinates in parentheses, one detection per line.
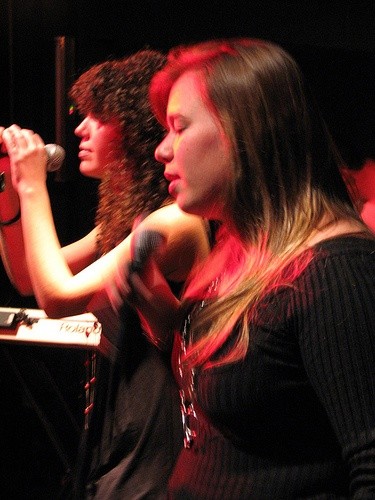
top-left (45, 143), bottom-right (66, 171)
top-left (115, 230), bottom-right (166, 380)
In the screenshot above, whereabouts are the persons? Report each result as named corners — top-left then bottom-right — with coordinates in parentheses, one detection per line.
top-left (0, 49), bottom-right (217, 500)
top-left (145, 34), bottom-right (375, 500)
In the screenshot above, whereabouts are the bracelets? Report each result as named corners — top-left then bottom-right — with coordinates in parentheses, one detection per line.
top-left (0, 208), bottom-right (21, 226)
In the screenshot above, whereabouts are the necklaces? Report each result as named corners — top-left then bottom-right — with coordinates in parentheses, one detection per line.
top-left (178, 272), bottom-right (227, 450)
